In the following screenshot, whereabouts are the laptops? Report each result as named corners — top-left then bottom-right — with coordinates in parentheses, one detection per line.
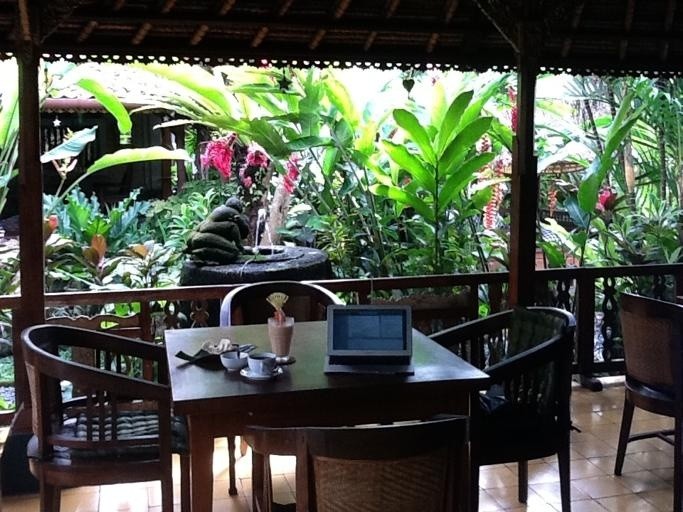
top-left (324, 304), bottom-right (414, 375)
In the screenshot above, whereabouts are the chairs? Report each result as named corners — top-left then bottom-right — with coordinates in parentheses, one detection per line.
top-left (220, 281), bottom-right (348, 496)
top-left (19, 323), bottom-right (174, 512)
top-left (427, 306), bottom-right (579, 512)
top-left (240, 410), bottom-right (472, 512)
top-left (613, 285), bottom-right (683, 512)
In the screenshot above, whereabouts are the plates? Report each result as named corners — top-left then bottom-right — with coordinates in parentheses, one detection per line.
top-left (237, 366), bottom-right (285, 381)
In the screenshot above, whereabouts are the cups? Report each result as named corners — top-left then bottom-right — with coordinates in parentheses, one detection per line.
top-left (247, 351), bottom-right (278, 375)
top-left (266, 316), bottom-right (298, 364)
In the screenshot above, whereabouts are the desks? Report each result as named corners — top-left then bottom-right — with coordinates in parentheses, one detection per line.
top-left (163, 320), bottom-right (493, 511)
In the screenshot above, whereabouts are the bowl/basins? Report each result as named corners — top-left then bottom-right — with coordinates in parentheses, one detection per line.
top-left (220, 350), bottom-right (249, 374)
